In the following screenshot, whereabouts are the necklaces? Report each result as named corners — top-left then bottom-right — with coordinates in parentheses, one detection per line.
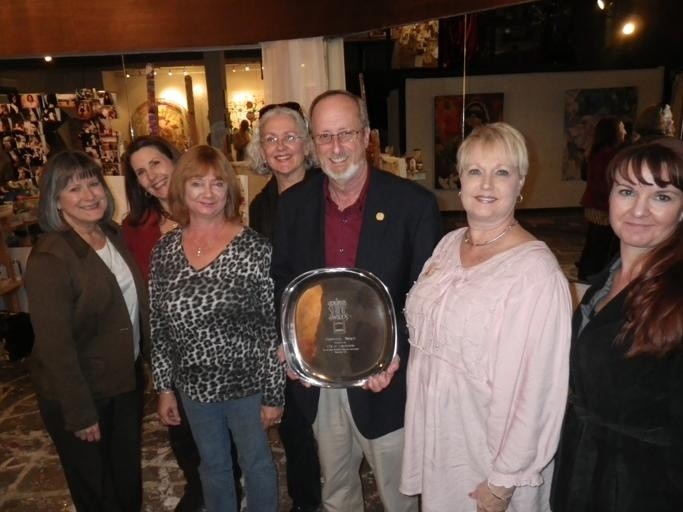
top-left (186, 222), bottom-right (226, 257)
top-left (464, 220), bottom-right (518, 247)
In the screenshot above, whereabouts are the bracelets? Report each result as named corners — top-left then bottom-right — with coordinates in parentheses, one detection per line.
top-left (486, 479), bottom-right (513, 504)
top-left (159, 391), bottom-right (174, 395)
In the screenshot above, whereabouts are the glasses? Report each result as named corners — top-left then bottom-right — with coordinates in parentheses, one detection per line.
top-left (260, 134), bottom-right (305, 146)
top-left (315, 126), bottom-right (367, 143)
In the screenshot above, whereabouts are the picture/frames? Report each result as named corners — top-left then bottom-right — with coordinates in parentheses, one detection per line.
top-left (399, 62), bottom-right (671, 218)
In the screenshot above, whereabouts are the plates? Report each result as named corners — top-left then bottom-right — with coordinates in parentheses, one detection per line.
top-left (280, 267), bottom-right (398, 387)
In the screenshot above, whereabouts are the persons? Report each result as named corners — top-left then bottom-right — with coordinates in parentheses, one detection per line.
top-left (24, 149), bottom-right (149, 512)
top-left (270, 90), bottom-right (441, 512)
top-left (121, 135), bottom-right (243, 512)
top-left (398, 122), bottom-right (573, 512)
top-left (579, 116), bottom-right (628, 286)
top-left (635, 102), bottom-right (676, 143)
top-left (147, 144), bottom-right (286, 512)
top-left (232, 120), bottom-right (251, 161)
top-left (549, 139), bottom-right (683, 512)
top-left (251, 108), bottom-right (326, 512)
